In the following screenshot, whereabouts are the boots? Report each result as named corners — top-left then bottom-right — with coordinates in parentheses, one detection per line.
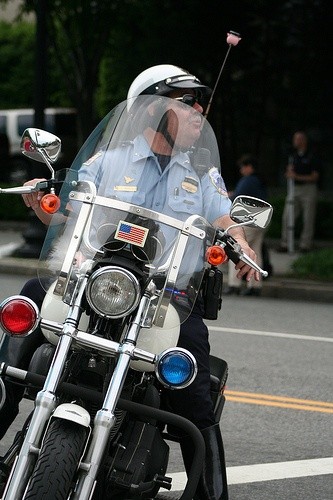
top-left (178, 423), bottom-right (229, 500)
top-left (0, 326), bottom-right (48, 440)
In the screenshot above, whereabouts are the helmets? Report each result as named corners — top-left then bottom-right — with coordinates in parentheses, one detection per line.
top-left (127, 65), bottom-right (201, 134)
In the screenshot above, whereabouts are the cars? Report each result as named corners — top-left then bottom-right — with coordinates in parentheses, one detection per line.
top-left (0, 106), bottom-right (80, 155)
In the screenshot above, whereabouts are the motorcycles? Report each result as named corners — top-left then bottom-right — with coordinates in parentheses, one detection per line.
top-left (0, 95), bottom-right (274, 496)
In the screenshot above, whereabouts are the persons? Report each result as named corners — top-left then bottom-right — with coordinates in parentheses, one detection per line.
top-left (277, 132), bottom-right (319, 252)
top-left (22, 65), bottom-right (259, 500)
top-left (227, 155), bottom-right (273, 281)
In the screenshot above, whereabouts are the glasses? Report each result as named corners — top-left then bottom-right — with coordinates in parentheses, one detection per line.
top-left (174, 94), bottom-right (201, 108)
